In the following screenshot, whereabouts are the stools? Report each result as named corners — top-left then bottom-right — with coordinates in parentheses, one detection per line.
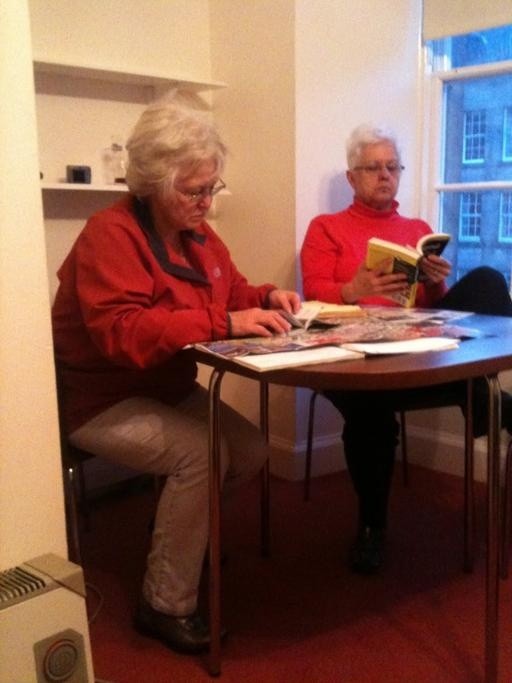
top-left (60, 422), bottom-right (162, 569)
top-left (301, 385), bottom-right (466, 500)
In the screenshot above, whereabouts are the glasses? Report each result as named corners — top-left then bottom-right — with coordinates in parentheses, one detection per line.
top-left (353, 165), bottom-right (405, 175)
top-left (172, 178), bottom-right (226, 203)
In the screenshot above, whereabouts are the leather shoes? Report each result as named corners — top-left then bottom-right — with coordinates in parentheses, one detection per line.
top-left (132, 606), bottom-right (231, 656)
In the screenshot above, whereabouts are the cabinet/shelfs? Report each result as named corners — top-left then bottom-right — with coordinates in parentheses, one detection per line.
top-left (32, 53), bottom-right (234, 200)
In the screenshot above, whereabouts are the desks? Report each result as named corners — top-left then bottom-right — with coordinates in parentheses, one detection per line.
top-left (189, 304), bottom-right (511, 681)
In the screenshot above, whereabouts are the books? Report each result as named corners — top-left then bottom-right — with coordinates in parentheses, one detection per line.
top-left (195, 301), bottom-right (482, 374)
top-left (366, 232), bottom-right (450, 308)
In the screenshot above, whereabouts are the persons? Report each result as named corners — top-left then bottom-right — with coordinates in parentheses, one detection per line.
top-left (53, 102), bottom-right (300, 652)
top-left (300, 124), bottom-right (511, 574)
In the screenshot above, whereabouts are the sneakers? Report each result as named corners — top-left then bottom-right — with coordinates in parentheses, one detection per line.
top-left (349, 525), bottom-right (388, 575)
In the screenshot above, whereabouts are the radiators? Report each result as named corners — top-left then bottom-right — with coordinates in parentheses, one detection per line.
top-left (1, 553), bottom-right (100, 683)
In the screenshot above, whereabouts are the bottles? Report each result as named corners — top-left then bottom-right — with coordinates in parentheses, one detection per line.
top-left (108, 142), bottom-right (126, 184)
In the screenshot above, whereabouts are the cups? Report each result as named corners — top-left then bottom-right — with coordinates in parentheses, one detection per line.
top-left (65, 165), bottom-right (92, 182)
